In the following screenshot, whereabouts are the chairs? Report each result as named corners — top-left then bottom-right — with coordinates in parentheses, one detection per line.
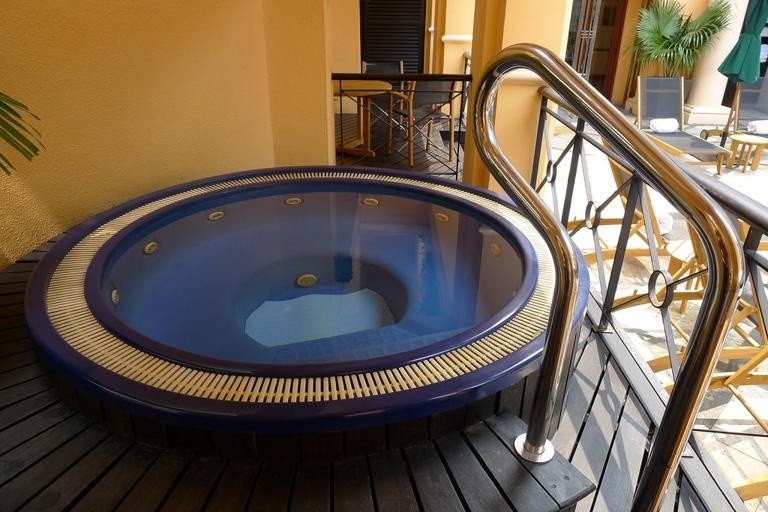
top-left (359, 58), bottom-right (456, 167)
top-left (647, 214), bottom-right (768, 437)
top-left (634, 74), bottom-right (732, 177)
top-left (565, 134), bottom-right (708, 315)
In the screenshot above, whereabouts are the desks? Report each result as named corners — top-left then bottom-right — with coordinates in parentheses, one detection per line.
top-left (331, 82), bottom-right (393, 159)
top-left (727, 134), bottom-right (768, 173)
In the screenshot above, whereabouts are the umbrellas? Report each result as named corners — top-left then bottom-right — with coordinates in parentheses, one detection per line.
top-left (717, 0), bottom-right (768, 131)
